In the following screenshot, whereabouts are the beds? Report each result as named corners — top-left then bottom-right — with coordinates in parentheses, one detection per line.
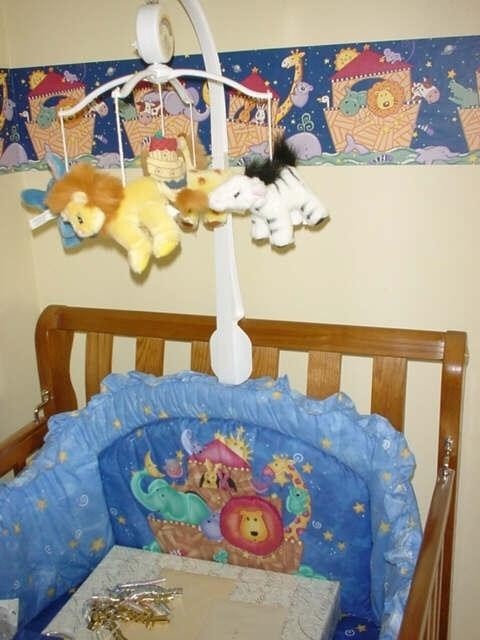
top-left (3, 305), bottom-right (470, 640)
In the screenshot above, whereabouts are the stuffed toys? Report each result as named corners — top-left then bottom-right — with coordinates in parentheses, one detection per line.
top-left (22, 151), bottom-right (99, 249)
top-left (44, 161), bottom-right (181, 275)
top-left (209, 151), bottom-right (328, 249)
top-left (173, 188), bottom-right (227, 229)
